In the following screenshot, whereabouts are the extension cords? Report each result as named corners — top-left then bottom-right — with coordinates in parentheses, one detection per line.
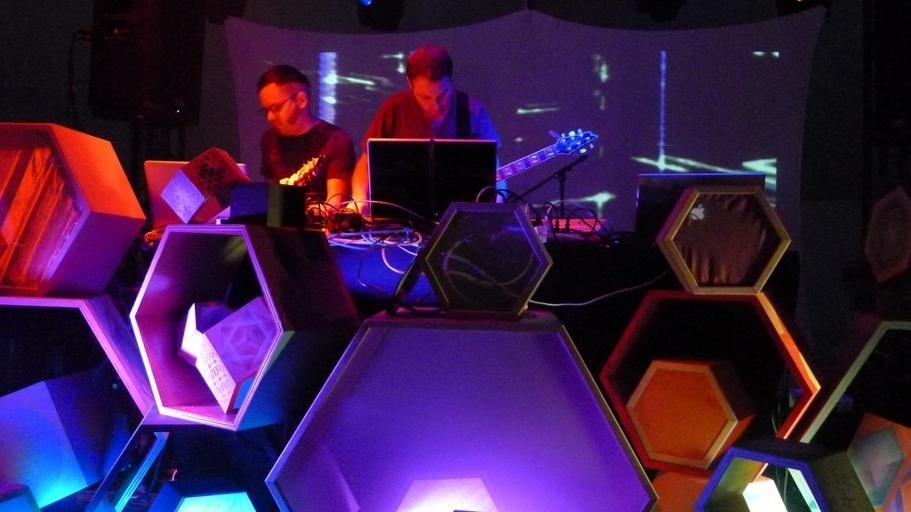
top-left (541, 232), bottom-right (601, 246)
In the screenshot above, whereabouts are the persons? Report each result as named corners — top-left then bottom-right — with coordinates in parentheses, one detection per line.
top-left (346, 46), bottom-right (507, 215)
top-left (255, 64), bottom-right (354, 215)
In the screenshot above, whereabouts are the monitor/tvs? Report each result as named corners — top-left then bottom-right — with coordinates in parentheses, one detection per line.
top-left (366, 138), bottom-right (497, 226)
top-left (631, 172), bottom-right (767, 242)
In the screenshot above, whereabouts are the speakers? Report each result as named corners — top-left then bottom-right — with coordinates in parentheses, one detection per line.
top-left (228, 183), bottom-right (306, 229)
